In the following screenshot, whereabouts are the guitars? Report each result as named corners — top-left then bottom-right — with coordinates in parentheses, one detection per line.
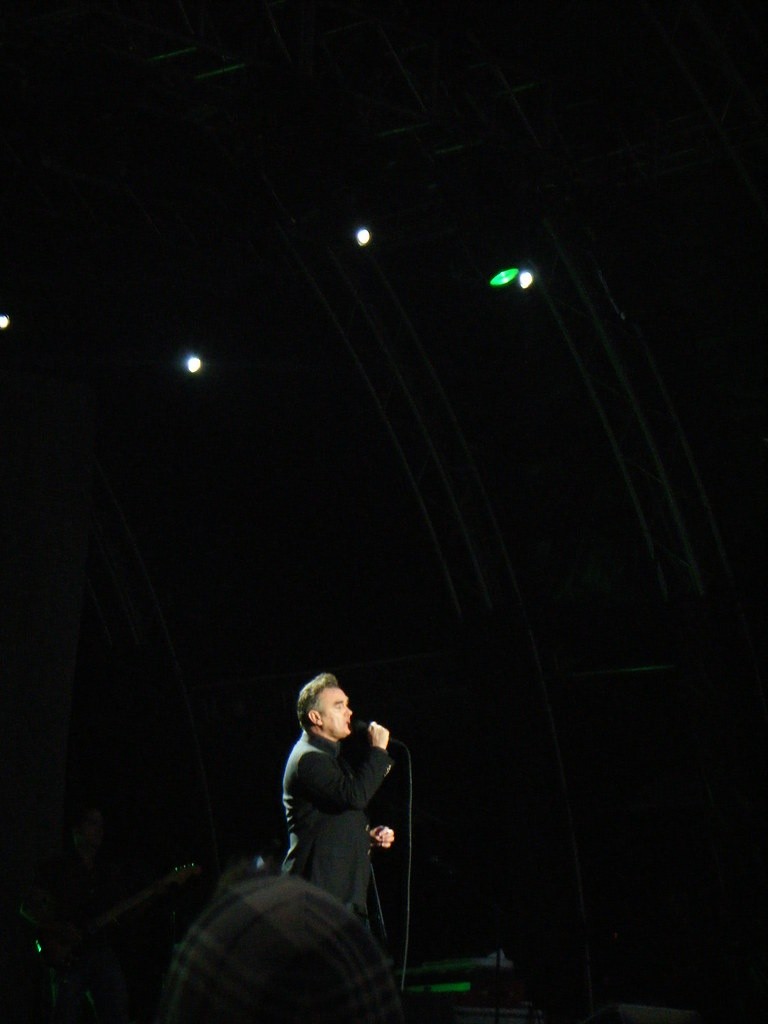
top-left (34, 862), bottom-right (200, 970)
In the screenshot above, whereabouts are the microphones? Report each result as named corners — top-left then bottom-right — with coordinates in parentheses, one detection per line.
top-left (355, 722), bottom-right (404, 747)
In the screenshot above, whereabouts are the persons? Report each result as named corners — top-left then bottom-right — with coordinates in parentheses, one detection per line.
top-left (282, 674), bottom-right (395, 930)
top-left (158, 831), bottom-right (405, 1024)
top-left (20, 807), bottom-right (165, 1024)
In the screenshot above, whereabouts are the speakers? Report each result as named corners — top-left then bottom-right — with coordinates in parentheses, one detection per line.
top-left (585, 1002), bottom-right (701, 1024)
